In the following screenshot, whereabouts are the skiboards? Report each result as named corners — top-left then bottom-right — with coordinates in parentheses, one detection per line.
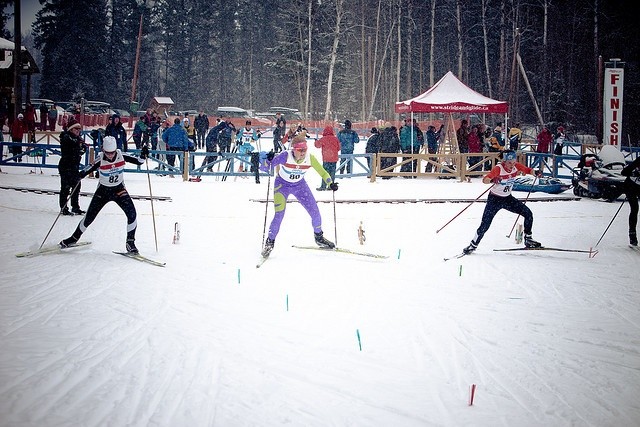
top-left (256, 244), bottom-right (390, 268)
top-left (443, 247), bottom-right (597, 261)
top-left (10, 241), bottom-right (166, 268)
top-left (626, 245), bottom-right (640, 257)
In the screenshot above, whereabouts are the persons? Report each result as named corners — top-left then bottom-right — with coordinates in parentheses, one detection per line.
top-left (132, 108), bottom-right (161, 159)
top-left (457, 119), bottom-right (505, 178)
top-left (365, 117), bottom-right (424, 178)
top-left (108, 114), bottom-right (127, 152)
top-left (182, 118), bottom-right (196, 169)
top-left (315, 126), bottom-right (340, 190)
top-left (157, 119), bottom-right (168, 164)
top-left (58, 119), bottom-right (88, 215)
top-left (537, 127), bottom-right (551, 164)
top-left (463, 151), bottom-right (541, 254)
top-left (48, 105), bottom-right (57, 130)
top-left (273, 120), bottom-right (286, 152)
top-left (60, 136), bottom-right (149, 254)
top-left (282, 123), bottom-right (299, 143)
top-left (24, 100), bottom-right (37, 131)
top-left (180, 112), bottom-right (191, 126)
top-left (11, 113), bottom-right (24, 162)
top-left (40, 101), bottom-right (48, 130)
top-left (550, 126), bottom-right (565, 167)
top-left (509, 123), bottom-right (521, 150)
top-left (337, 119), bottom-right (359, 178)
top-left (425, 125), bottom-right (440, 171)
top-left (276, 112), bottom-right (286, 125)
top-left (216, 115), bottom-right (236, 160)
top-left (296, 124), bottom-right (310, 138)
top-left (621, 156), bottom-right (640, 246)
top-left (105, 116), bottom-right (113, 135)
top-left (194, 110), bottom-right (209, 148)
top-left (162, 118), bottom-right (188, 178)
top-left (199, 122), bottom-right (226, 171)
top-left (237, 120), bottom-right (260, 172)
top-left (260, 136), bottom-right (338, 256)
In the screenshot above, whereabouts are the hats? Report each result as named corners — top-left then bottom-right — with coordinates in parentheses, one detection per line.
top-left (559, 125), bottom-right (564, 131)
top-left (247, 121), bottom-right (251, 125)
top-left (183, 118), bottom-right (189, 122)
top-left (67, 119), bottom-right (80, 130)
top-left (158, 118), bottom-right (166, 124)
top-left (174, 118), bottom-right (180, 123)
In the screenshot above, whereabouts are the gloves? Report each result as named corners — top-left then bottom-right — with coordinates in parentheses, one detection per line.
top-left (329, 183), bottom-right (339, 191)
top-left (76, 169), bottom-right (87, 180)
top-left (533, 170), bottom-right (542, 178)
top-left (140, 145), bottom-right (150, 158)
top-left (259, 134), bottom-right (262, 137)
top-left (632, 170), bottom-right (639, 176)
top-left (266, 149), bottom-right (276, 161)
top-left (491, 176), bottom-right (502, 185)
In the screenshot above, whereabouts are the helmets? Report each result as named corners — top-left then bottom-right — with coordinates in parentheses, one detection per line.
top-left (102, 135), bottom-right (118, 152)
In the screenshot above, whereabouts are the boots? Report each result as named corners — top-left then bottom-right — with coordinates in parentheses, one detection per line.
top-left (525, 231), bottom-right (541, 248)
top-left (314, 231), bottom-right (335, 248)
top-left (262, 238), bottom-right (274, 257)
top-left (463, 239), bottom-right (477, 254)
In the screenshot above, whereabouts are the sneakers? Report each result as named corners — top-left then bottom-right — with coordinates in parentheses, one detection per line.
top-left (238, 165), bottom-right (243, 172)
top-left (60, 236), bottom-right (76, 246)
top-left (127, 240), bottom-right (138, 252)
top-left (61, 208), bottom-right (74, 215)
top-left (629, 230), bottom-right (638, 246)
top-left (316, 187), bottom-right (326, 191)
top-left (72, 209), bottom-right (85, 214)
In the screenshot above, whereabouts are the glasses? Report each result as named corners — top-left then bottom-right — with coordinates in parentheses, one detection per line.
top-left (508, 161), bottom-right (516, 164)
top-left (185, 121), bottom-right (189, 122)
top-left (73, 127), bottom-right (80, 131)
top-left (297, 148), bottom-right (307, 152)
top-left (161, 121), bottom-right (165, 123)
